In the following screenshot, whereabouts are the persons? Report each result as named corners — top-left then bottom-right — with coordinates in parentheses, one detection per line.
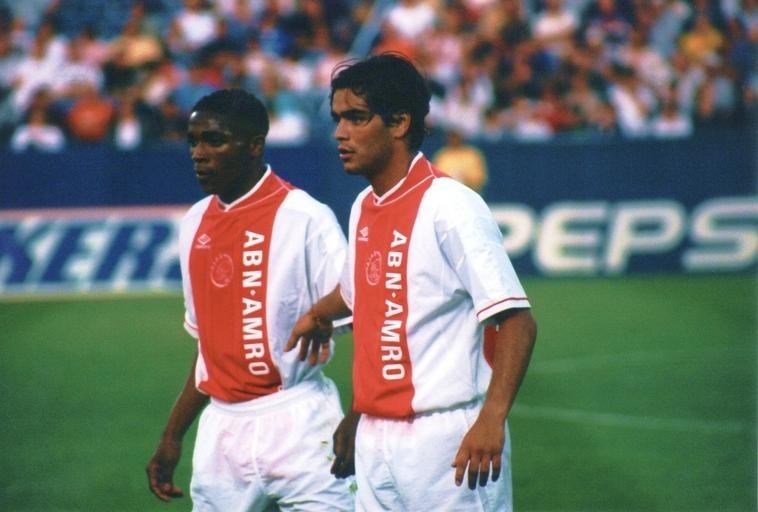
top-left (145, 88), bottom-right (356, 512)
top-left (283, 50), bottom-right (537, 512)
top-left (1, 2), bottom-right (757, 151)
